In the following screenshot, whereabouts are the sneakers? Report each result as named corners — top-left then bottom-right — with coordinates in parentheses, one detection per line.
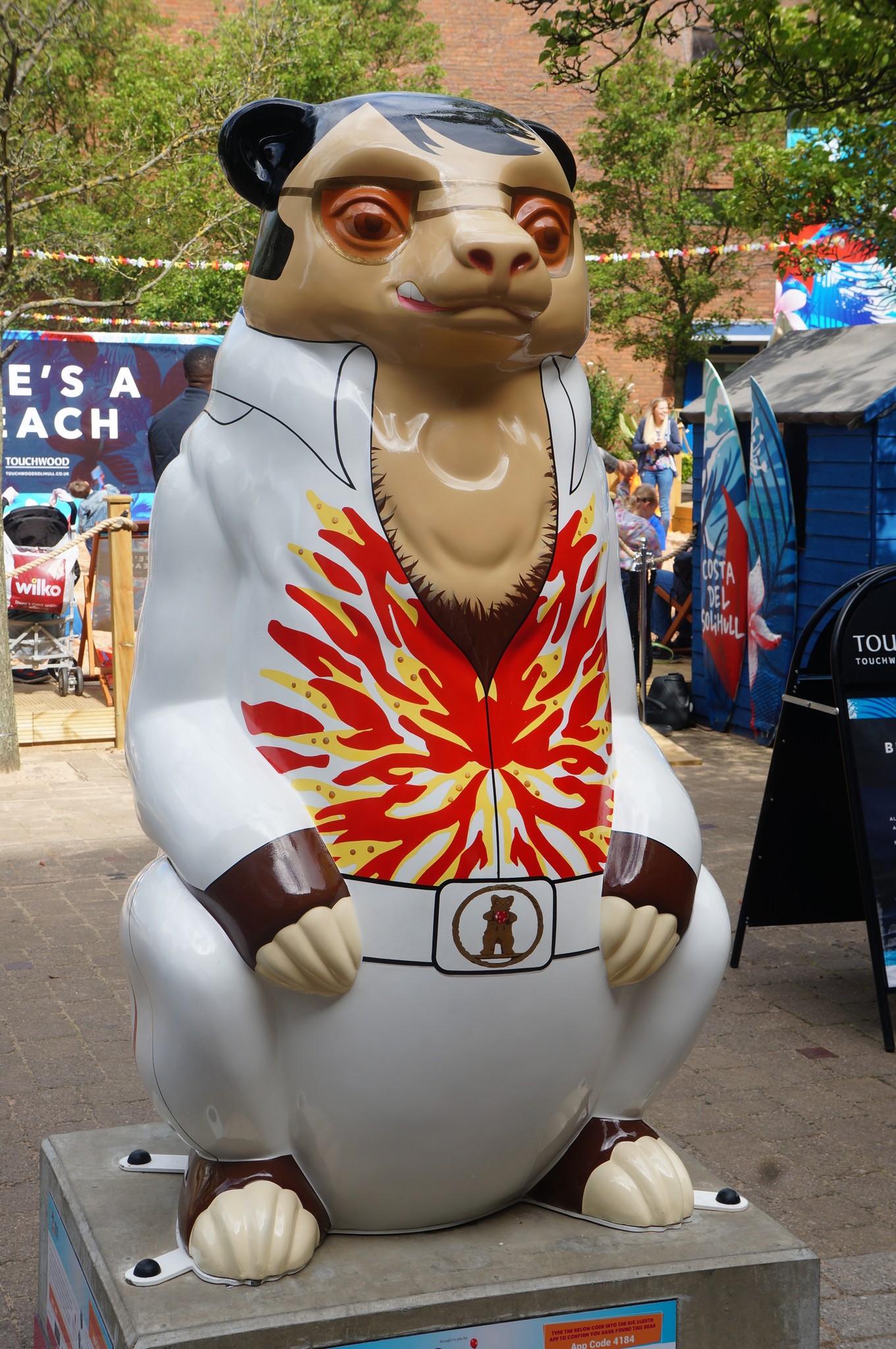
top-left (12, 669), bottom-right (51, 683)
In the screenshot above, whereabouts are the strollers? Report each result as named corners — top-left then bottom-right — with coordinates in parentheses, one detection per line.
top-left (1, 485), bottom-right (86, 697)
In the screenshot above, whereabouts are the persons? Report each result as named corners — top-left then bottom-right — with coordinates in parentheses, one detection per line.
top-left (599, 395), bottom-right (691, 690)
top-left (147, 343), bottom-right (216, 484)
top-left (68, 481), bottom-right (120, 584)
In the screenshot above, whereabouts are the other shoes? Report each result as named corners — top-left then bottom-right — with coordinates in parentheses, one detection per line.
top-left (652, 646), bottom-right (685, 663)
top-left (672, 638), bottom-right (692, 657)
